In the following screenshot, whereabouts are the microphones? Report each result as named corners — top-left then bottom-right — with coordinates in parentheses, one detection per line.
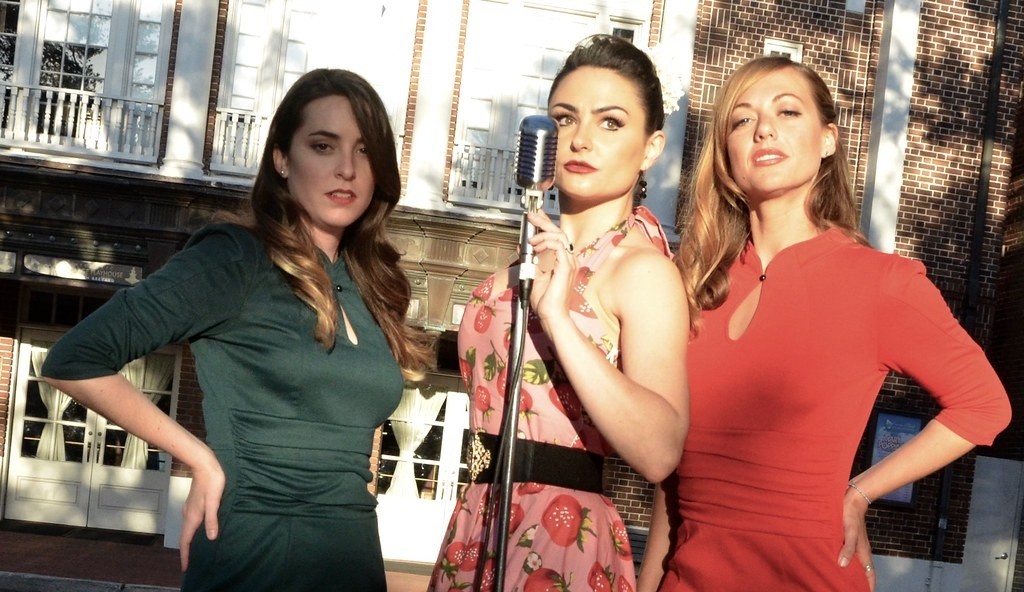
top-left (514, 116), bottom-right (560, 310)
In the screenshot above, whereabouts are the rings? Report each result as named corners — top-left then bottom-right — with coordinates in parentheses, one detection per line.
top-left (864, 562), bottom-right (874, 573)
top-left (565, 242), bottom-right (574, 254)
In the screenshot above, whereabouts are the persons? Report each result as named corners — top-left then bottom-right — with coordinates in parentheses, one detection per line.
top-left (639, 57), bottom-right (1012, 592)
top-left (40, 68), bottom-right (436, 592)
top-left (427, 36), bottom-right (689, 592)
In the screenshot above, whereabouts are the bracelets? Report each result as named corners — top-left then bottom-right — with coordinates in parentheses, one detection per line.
top-left (847, 481), bottom-right (872, 504)
